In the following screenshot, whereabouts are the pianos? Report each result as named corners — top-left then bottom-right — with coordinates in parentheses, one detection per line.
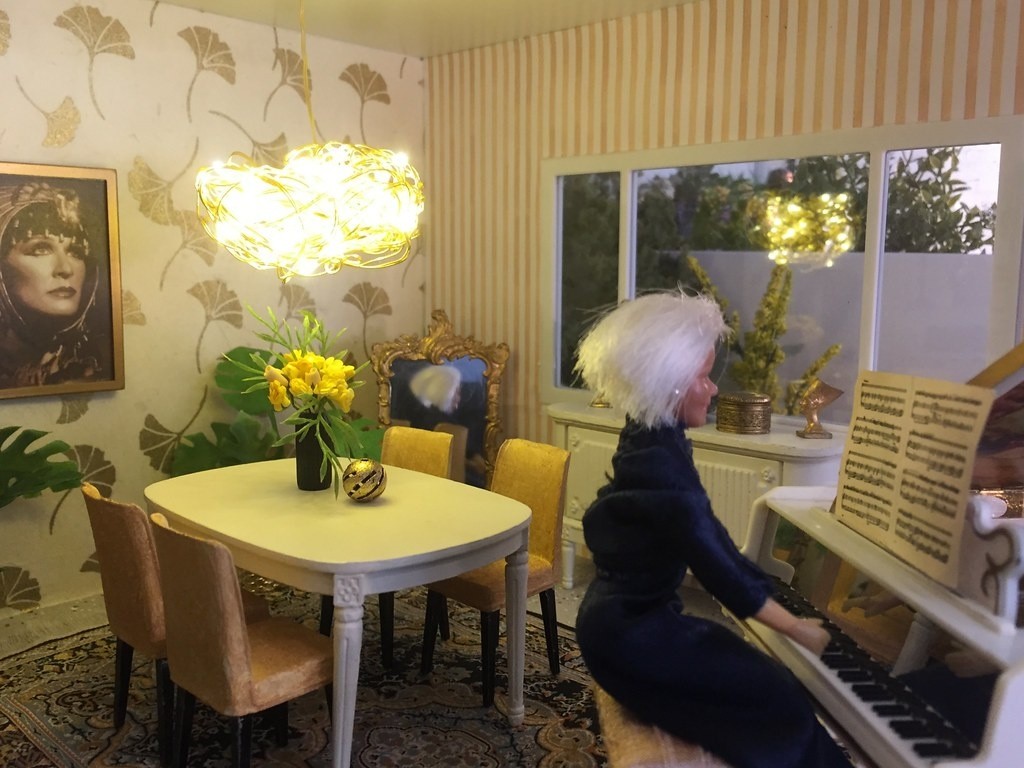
top-left (716, 485), bottom-right (1023, 768)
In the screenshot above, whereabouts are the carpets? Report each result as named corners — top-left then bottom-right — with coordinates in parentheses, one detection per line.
top-left (0, 582), bottom-right (611, 768)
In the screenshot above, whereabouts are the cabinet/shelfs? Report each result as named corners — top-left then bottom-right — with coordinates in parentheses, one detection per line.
top-left (548, 400), bottom-right (849, 589)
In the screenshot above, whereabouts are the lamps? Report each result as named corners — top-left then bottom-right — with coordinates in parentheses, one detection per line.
top-left (194, 0), bottom-right (423, 276)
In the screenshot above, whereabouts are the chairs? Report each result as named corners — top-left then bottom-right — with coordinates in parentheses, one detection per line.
top-left (423, 438), bottom-right (573, 703)
top-left (318, 424), bottom-right (454, 668)
top-left (81, 482), bottom-right (271, 768)
top-left (149, 511), bottom-right (334, 768)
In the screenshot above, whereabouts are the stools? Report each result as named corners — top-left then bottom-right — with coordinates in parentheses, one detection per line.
top-left (592, 676), bottom-right (736, 768)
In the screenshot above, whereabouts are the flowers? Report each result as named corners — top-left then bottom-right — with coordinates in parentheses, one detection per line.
top-left (217, 295), bottom-right (371, 503)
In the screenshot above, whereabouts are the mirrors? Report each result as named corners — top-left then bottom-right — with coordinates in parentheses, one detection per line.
top-left (371, 309), bottom-right (510, 491)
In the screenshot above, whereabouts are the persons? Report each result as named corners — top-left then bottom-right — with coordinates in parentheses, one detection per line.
top-left (572, 280), bottom-right (856, 768)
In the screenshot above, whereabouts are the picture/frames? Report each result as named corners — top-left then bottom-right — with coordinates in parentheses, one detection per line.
top-left (0, 161), bottom-right (126, 400)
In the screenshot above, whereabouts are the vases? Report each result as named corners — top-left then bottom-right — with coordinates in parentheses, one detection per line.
top-left (295, 412), bottom-right (332, 491)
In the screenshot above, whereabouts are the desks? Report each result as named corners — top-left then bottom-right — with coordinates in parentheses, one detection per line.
top-left (143, 456), bottom-right (531, 768)
